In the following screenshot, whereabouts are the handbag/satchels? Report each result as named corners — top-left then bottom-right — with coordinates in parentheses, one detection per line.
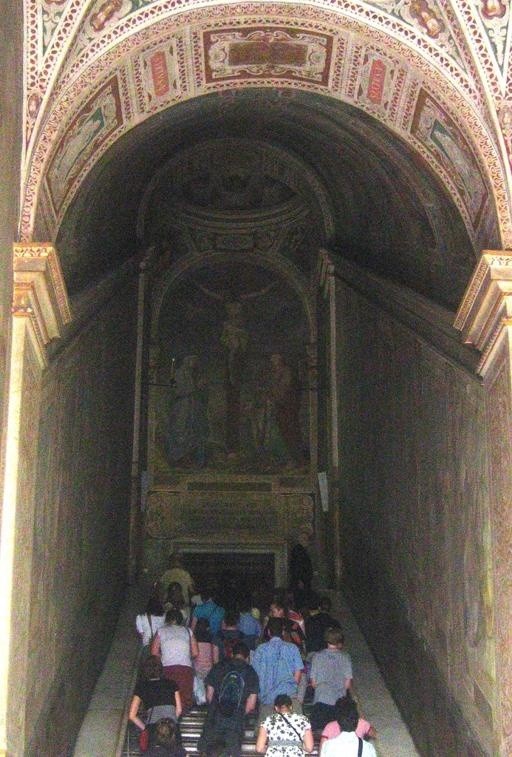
top-left (193, 676), bottom-right (206, 706)
top-left (194, 617), bottom-right (212, 642)
top-left (138, 730), bottom-right (149, 751)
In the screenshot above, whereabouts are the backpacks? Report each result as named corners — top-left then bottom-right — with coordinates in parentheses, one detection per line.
top-left (218, 659), bottom-right (253, 718)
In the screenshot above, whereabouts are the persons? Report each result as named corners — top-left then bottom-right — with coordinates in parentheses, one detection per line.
top-left (287, 532), bottom-right (313, 591)
top-left (158, 560), bottom-right (197, 605)
top-left (169, 301), bottom-right (307, 475)
